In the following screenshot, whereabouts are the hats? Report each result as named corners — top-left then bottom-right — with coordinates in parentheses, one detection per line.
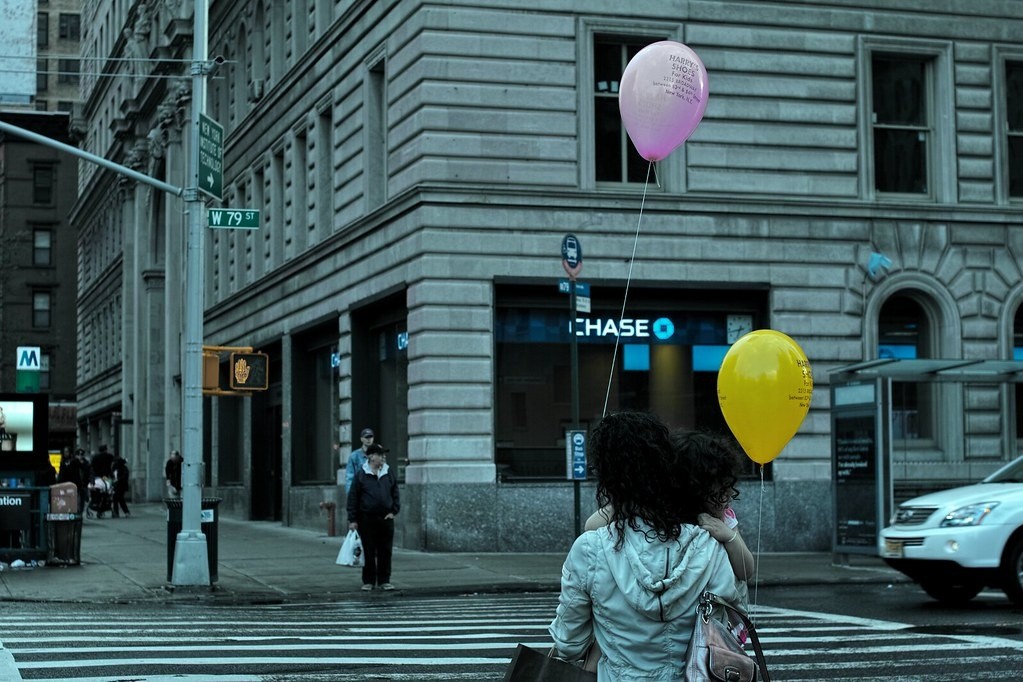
top-left (366, 444), bottom-right (390, 455)
top-left (361, 429), bottom-right (375, 437)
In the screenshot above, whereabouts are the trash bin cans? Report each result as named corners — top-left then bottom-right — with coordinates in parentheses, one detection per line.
top-left (162, 497), bottom-right (222, 583)
top-left (44, 514), bottom-right (82, 565)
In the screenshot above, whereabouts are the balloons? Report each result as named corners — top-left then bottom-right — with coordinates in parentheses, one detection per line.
top-left (618, 40), bottom-right (708, 161)
top-left (717, 329), bottom-right (814, 464)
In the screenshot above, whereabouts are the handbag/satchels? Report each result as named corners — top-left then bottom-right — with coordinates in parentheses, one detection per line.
top-left (336, 530), bottom-right (365, 567)
top-left (686, 590), bottom-right (770, 682)
top-left (502, 643), bottom-right (598, 682)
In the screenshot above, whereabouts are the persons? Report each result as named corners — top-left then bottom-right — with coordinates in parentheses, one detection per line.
top-left (548, 413), bottom-right (757, 682)
top-left (347, 444), bottom-right (399, 591)
top-left (59, 445), bottom-right (131, 519)
top-left (165, 451), bottom-right (183, 496)
top-left (345, 429), bottom-right (374, 493)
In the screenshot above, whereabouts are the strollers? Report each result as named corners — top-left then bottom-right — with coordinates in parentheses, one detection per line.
top-left (85, 480), bottom-right (118, 520)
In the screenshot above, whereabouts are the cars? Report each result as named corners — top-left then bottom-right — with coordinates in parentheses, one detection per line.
top-left (878, 453), bottom-right (1023, 609)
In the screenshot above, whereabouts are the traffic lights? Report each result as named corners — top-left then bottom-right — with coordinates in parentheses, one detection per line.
top-left (230, 352), bottom-right (269, 390)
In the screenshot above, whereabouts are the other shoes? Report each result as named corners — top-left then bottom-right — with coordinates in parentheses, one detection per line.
top-left (361, 584), bottom-right (372, 591)
top-left (381, 583), bottom-right (395, 590)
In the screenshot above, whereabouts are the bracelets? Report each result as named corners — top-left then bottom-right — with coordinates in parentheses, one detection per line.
top-left (729, 532), bottom-right (737, 542)
